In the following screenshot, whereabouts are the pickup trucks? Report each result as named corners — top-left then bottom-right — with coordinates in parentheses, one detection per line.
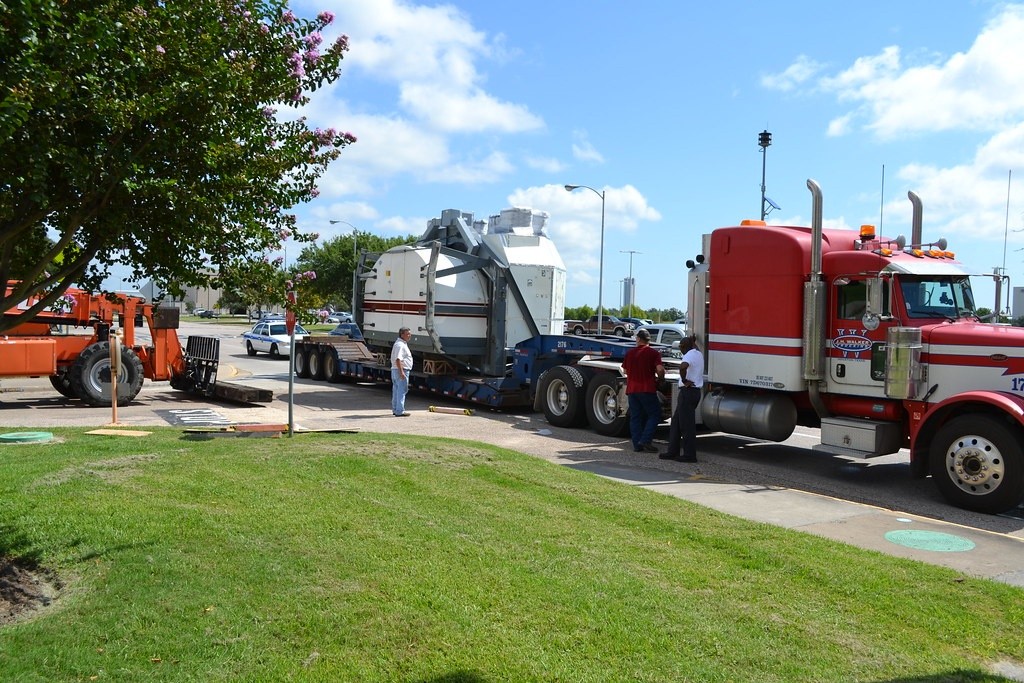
top-left (568, 315), bottom-right (635, 338)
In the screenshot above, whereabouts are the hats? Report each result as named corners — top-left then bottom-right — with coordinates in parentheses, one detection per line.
top-left (637, 329), bottom-right (651, 340)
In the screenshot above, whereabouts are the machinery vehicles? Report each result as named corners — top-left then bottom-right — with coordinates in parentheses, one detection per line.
top-left (0, 280), bottom-right (273, 408)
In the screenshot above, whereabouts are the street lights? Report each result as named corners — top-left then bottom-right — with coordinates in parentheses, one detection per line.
top-left (564, 184), bottom-right (605, 335)
top-left (330, 220), bottom-right (357, 323)
top-left (619, 251), bottom-right (644, 318)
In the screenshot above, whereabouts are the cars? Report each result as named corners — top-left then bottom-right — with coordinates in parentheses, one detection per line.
top-left (194, 308), bottom-right (206, 316)
top-left (243, 320), bottom-right (312, 360)
top-left (200, 311), bottom-right (218, 319)
top-left (250, 310), bottom-right (272, 319)
top-left (619, 318), bottom-right (650, 329)
top-left (327, 318), bottom-right (339, 324)
top-left (330, 312), bottom-right (353, 323)
top-left (633, 324), bottom-right (687, 354)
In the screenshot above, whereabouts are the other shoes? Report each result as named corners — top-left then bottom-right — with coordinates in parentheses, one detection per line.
top-left (396, 412), bottom-right (410, 417)
top-left (393, 411), bottom-right (396, 415)
top-left (659, 452), bottom-right (680, 460)
top-left (633, 445), bottom-right (643, 452)
top-left (638, 441), bottom-right (658, 453)
top-left (674, 455), bottom-right (697, 463)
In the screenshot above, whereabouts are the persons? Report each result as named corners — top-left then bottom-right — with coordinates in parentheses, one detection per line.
top-left (390, 326), bottom-right (414, 417)
top-left (621, 328), bottom-right (666, 453)
top-left (658, 333), bottom-right (705, 463)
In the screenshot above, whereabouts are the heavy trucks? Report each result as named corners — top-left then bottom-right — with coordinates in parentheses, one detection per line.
top-left (296, 179), bottom-right (1023, 515)
